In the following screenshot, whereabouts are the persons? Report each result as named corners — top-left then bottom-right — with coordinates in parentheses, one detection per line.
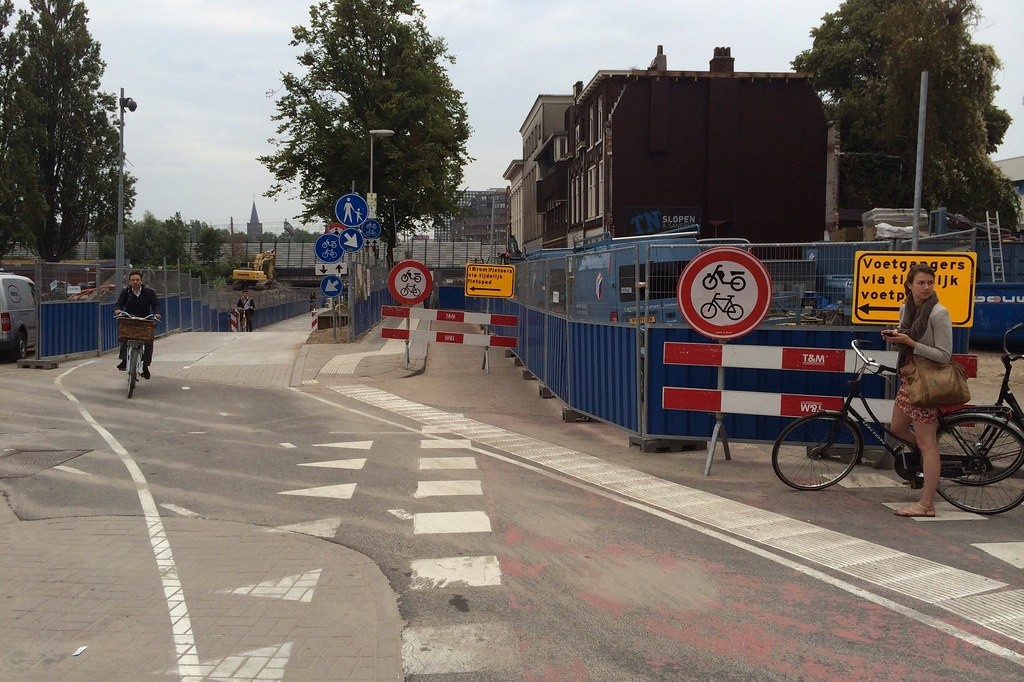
top-left (236, 291), bottom-right (256, 331)
top-left (112, 271), bottom-right (162, 380)
top-left (882, 262), bottom-right (955, 518)
top-left (309, 291), bottom-right (318, 311)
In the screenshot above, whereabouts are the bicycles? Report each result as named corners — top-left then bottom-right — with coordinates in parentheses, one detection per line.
top-left (936, 322), bottom-right (1024, 488)
top-left (237, 306), bottom-right (253, 332)
top-left (112, 310), bottom-right (162, 398)
top-left (309, 299), bottom-right (317, 313)
top-left (771, 339), bottom-right (1024, 516)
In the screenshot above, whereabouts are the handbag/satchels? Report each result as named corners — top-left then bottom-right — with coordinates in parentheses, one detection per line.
top-left (899, 360), bottom-right (972, 404)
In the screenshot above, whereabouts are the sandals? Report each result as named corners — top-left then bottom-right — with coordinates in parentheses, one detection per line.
top-left (893, 501), bottom-right (935, 517)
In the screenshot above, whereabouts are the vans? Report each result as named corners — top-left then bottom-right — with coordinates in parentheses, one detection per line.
top-left (0, 270), bottom-right (37, 361)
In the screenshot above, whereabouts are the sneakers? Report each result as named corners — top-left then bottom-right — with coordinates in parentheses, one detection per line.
top-left (116, 360), bottom-right (126, 369)
top-left (142, 365), bottom-right (151, 379)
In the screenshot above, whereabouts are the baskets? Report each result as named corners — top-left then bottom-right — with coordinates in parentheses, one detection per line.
top-left (116, 317), bottom-right (155, 343)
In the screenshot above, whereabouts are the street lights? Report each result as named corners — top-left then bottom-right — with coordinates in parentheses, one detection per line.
top-left (115, 87), bottom-right (138, 305)
top-left (370, 129), bottom-right (394, 194)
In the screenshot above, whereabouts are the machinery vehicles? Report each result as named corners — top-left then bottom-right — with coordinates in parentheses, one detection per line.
top-left (232, 248), bottom-right (278, 290)
